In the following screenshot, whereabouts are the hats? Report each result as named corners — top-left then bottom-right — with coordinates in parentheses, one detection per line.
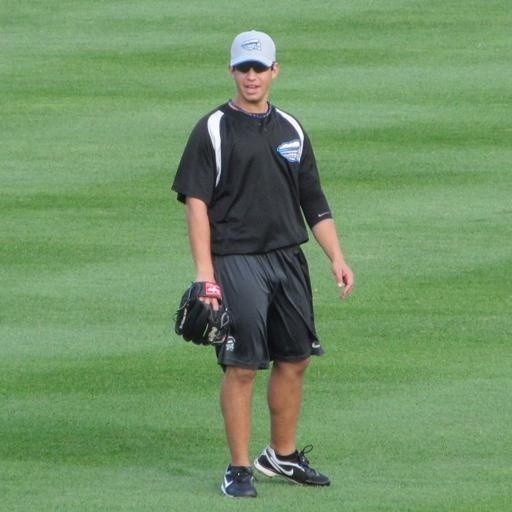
top-left (230, 30), bottom-right (276, 67)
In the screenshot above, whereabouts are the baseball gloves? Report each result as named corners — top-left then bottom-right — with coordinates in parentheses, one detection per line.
top-left (174, 282), bottom-right (229, 345)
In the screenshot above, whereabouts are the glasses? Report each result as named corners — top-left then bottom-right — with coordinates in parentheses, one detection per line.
top-left (236, 62), bottom-right (270, 73)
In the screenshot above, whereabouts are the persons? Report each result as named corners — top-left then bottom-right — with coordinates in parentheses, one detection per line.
top-left (170, 29), bottom-right (354, 498)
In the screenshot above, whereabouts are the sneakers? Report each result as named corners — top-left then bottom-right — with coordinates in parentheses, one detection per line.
top-left (220, 464), bottom-right (257, 499)
top-left (254, 445), bottom-right (331, 486)
